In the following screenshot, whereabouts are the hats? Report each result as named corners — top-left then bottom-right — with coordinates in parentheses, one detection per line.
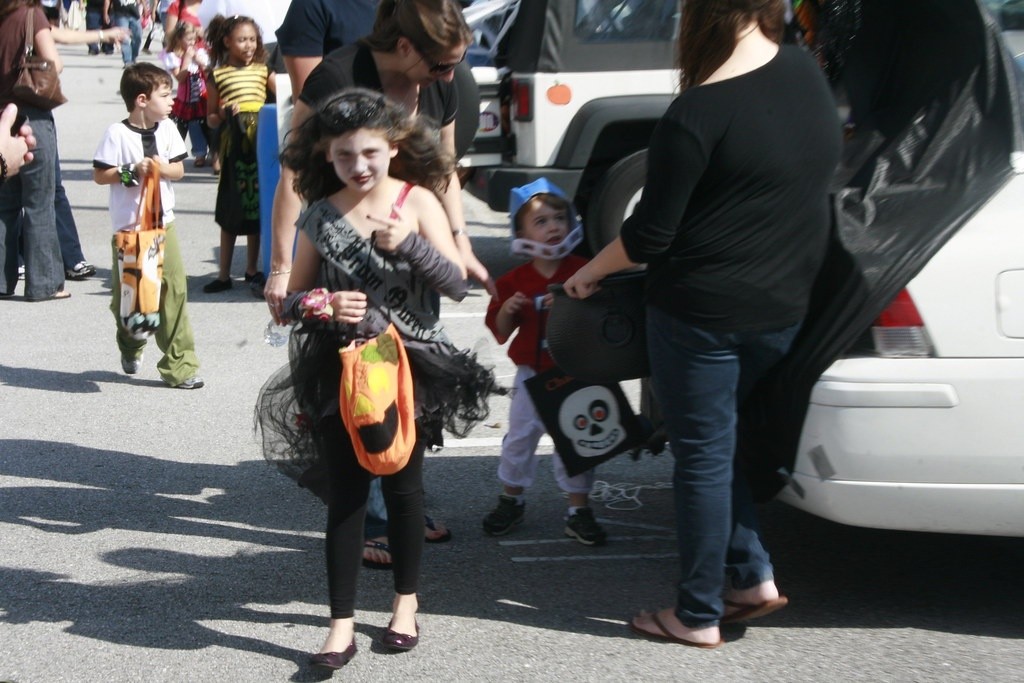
top-left (509, 178), bottom-right (584, 261)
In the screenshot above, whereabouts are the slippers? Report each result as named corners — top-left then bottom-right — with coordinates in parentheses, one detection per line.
top-left (720, 595), bottom-right (788, 624)
top-left (424, 515), bottom-right (452, 543)
top-left (362, 540), bottom-right (393, 570)
top-left (632, 607), bottom-right (724, 648)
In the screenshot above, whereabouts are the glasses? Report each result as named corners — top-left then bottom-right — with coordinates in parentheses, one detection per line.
top-left (413, 42), bottom-right (468, 76)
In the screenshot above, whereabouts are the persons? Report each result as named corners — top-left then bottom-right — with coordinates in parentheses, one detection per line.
top-left (562, 1), bottom-right (843, 650)
top-left (0, 0), bottom-right (134, 302)
top-left (92, 63), bottom-right (204, 388)
top-left (161, 0), bottom-right (222, 175)
top-left (480, 177), bottom-right (607, 546)
top-left (263, 0), bottom-right (499, 328)
top-left (280, 87), bottom-right (467, 669)
top-left (41, 0), bottom-right (159, 68)
top-left (202, 14), bottom-right (277, 293)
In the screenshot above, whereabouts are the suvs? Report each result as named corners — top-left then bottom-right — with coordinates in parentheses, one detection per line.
top-left (450, 0), bottom-right (1024, 537)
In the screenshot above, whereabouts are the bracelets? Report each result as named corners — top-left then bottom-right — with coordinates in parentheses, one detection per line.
top-left (117, 163), bottom-right (139, 188)
top-left (99, 29), bottom-right (103, 41)
top-left (267, 268), bottom-right (291, 274)
top-left (298, 287), bottom-right (334, 321)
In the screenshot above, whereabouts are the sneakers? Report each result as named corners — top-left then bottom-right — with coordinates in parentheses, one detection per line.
top-left (17, 265), bottom-right (26, 279)
top-left (481, 494), bottom-right (525, 535)
top-left (564, 508), bottom-right (606, 545)
top-left (160, 375), bottom-right (204, 388)
top-left (120, 353), bottom-right (143, 373)
top-left (66, 261), bottom-right (96, 278)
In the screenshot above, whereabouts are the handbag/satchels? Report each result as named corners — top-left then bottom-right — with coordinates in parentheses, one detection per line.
top-left (111, 161), bottom-right (166, 342)
top-left (521, 297), bottom-right (642, 477)
top-left (214, 105), bottom-right (260, 235)
top-left (12, 8), bottom-right (68, 111)
top-left (546, 271), bottom-right (647, 385)
top-left (338, 230), bottom-right (416, 474)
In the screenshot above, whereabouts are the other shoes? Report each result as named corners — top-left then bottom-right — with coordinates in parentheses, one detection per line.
top-left (88, 48), bottom-right (152, 69)
top-left (203, 278), bottom-right (231, 292)
top-left (0, 292), bottom-right (15, 298)
top-left (308, 636), bottom-right (357, 668)
top-left (244, 271), bottom-right (266, 283)
top-left (193, 160), bottom-right (205, 166)
top-left (384, 618), bottom-right (420, 649)
top-left (214, 168), bottom-right (221, 175)
top-left (29, 290), bottom-right (71, 301)
